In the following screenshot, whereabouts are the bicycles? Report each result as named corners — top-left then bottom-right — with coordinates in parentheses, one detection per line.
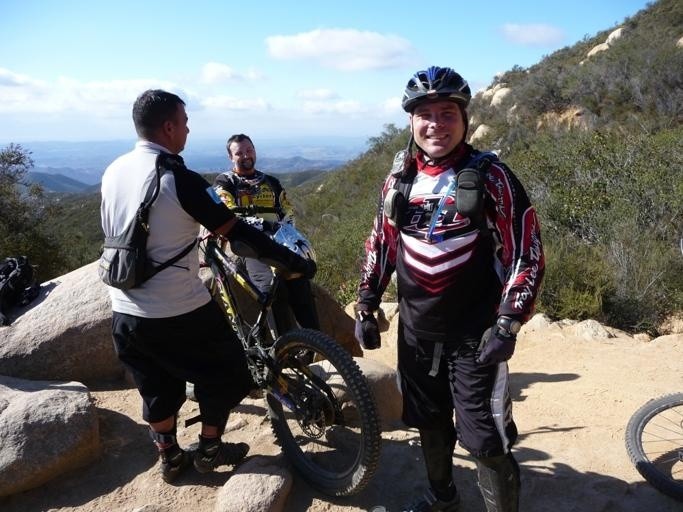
top-left (197, 203), bottom-right (381, 497)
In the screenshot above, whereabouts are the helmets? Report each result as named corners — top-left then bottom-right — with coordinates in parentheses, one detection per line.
top-left (402, 65), bottom-right (472, 112)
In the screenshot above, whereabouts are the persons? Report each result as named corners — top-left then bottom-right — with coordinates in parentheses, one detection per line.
top-left (350, 65), bottom-right (546, 510)
top-left (99, 87), bottom-right (255, 484)
top-left (211, 133), bottom-right (319, 365)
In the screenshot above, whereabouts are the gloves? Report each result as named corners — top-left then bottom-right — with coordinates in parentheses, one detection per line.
top-left (475, 317), bottom-right (521, 366)
top-left (353, 303), bottom-right (381, 350)
top-left (304, 259), bottom-right (317, 278)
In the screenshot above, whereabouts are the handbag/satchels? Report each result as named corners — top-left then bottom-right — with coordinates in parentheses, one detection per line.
top-left (98, 202), bottom-right (150, 290)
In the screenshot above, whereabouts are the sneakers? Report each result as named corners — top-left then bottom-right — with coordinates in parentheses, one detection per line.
top-left (160, 447), bottom-right (199, 482)
top-left (405, 489), bottom-right (460, 512)
top-left (195, 443), bottom-right (250, 473)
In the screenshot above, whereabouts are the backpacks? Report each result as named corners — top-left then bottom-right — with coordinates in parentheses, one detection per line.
top-left (0, 255), bottom-right (40, 326)
